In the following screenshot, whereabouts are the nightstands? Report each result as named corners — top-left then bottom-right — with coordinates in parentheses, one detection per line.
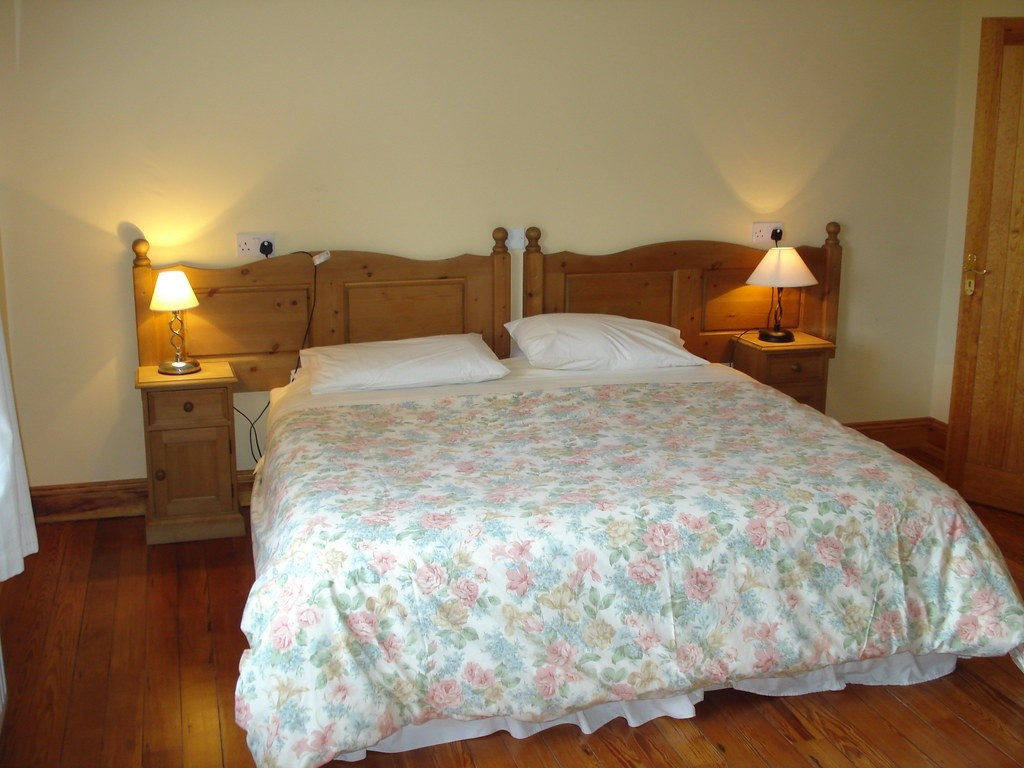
top-left (134, 363), bottom-right (247, 545)
top-left (730, 332), bottom-right (836, 414)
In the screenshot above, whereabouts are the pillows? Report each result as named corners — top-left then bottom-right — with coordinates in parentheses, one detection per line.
top-left (502, 311), bottom-right (711, 370)
top-left (300, 331), bottom-right (511, 397)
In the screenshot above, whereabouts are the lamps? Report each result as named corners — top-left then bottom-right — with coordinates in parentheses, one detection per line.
top-left (745, 246), bottom-right (820, 342)
top-left (150, 271), bottom-right (204, 373)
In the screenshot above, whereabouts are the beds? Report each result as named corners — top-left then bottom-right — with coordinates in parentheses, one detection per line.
top-left (123, 224), bottom-right (1024, 768)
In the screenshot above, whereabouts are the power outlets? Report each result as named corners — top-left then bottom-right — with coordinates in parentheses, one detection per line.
top-left (236, 231), bottom-right (278, 261)
top-left (752, 221), bottom-right (784, 242)
